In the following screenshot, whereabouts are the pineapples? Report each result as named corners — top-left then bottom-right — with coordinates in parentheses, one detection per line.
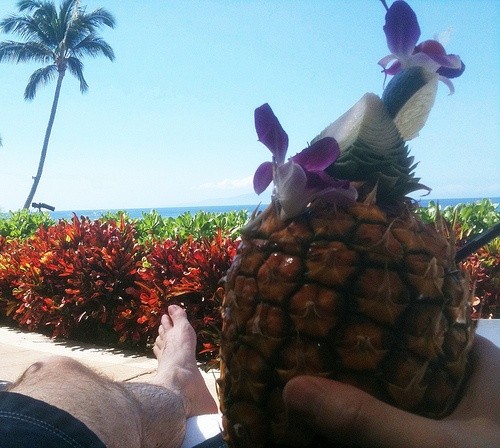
top-left (220, 95), bottom-right (477, 448)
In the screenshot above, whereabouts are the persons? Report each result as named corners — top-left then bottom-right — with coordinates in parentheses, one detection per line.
top-left (0, 301), bottom-right (499, 447)
